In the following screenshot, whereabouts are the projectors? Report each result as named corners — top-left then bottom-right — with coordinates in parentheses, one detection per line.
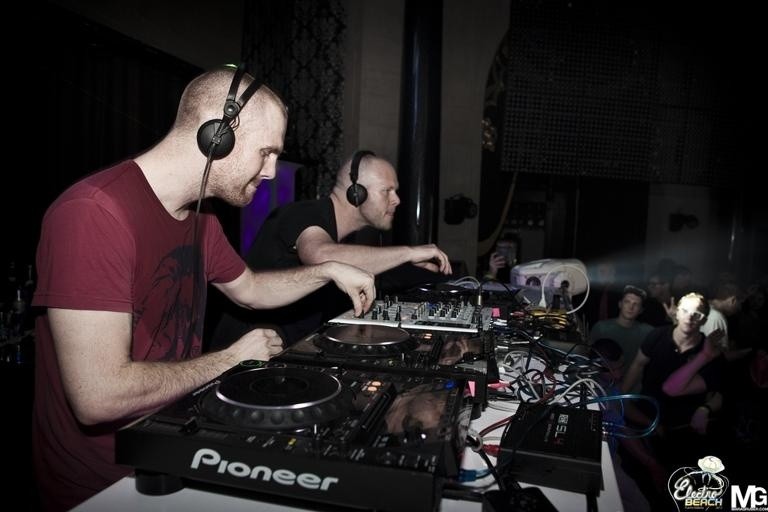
top-left (509, 258), bottom-right (591, 299)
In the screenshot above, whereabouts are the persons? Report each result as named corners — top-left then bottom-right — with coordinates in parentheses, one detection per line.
top-left (200, 149), bottom-right (453, 368)
top-left (23, 65), bottom-right (376, 511)
top-left (483, 234), bottom-right (766, 510)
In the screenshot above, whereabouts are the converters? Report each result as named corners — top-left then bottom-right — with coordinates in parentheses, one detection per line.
top-left (498, 398), bottom-right (604, 496)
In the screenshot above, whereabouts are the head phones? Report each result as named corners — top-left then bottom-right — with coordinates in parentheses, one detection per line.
top-left (197, 58), bottom-right (264, 158)
top-left (345, 148), bottom-right (377, 206)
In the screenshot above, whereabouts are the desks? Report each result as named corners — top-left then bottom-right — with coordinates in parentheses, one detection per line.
top-left (67, 332), bottom-right (633, 512)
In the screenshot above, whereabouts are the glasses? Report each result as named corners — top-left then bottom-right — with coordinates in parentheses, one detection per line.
top-left (623, 284), bottom-right (647, 297)
top-left (679, 306), bottom-right (706, 321)
